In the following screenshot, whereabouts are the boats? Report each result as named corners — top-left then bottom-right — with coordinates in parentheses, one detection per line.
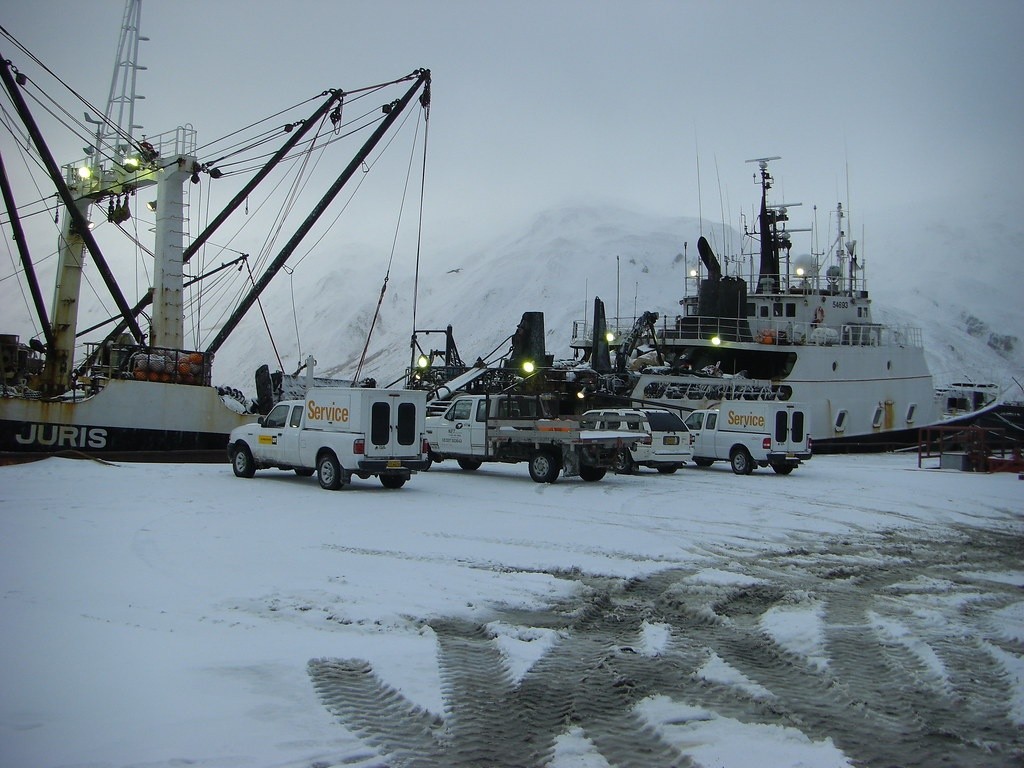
top-left (0, 0), bottom-right (1007, 465)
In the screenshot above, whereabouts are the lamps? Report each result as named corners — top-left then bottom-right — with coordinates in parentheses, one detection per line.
top-left (525, 361), bottom-right (535, 370)
top-left (712, 333), bottom-right (723, 346)
top-left (576, 387), bottom-right (587, 399)
top-left (147, 200), bottom-right (157, 210)
top-left (83, 146), bottom-right (95, 155)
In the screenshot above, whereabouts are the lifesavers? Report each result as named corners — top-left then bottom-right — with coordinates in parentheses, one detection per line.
top-left (679, 364), bottom-right (692, 371)
top-left (815, 306), bottom-right (824, 322)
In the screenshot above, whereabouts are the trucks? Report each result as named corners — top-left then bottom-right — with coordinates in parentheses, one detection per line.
top-left (424, 368), bottom-right (651, 482)
top-left (227, 386), bottom-right (427, 490)
top-left (684, 400), bottom-right (813, 475)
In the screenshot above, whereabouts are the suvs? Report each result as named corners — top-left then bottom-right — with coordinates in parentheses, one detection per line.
top-left (581, 406), bottom-right (695, 474)
